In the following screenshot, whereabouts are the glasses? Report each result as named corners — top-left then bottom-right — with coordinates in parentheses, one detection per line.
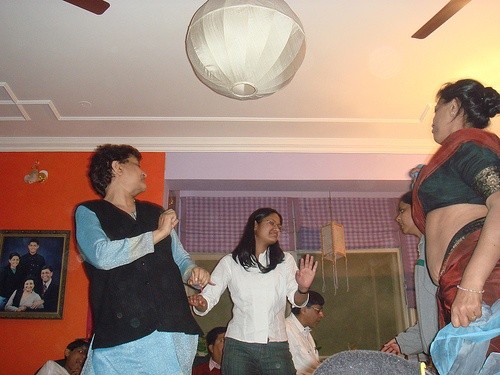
top-left (308, 305), bottom-right (321, 312)
top-left (259, 219), bottom-right (282, 232)
top-left (119, 162), bottom-right (141, 168)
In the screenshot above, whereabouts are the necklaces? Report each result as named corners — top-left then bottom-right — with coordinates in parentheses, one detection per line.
top-left (109, 194), bottom-right (137, 221)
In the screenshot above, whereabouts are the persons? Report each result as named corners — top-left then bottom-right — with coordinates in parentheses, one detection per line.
top-left (5, 275), bottom-right (44, 312)
top-left (35, 264), bottom-right (59, 312)
top-left (18, 239), bottom-right (45, 276)
top-left (1, 251), bottom-right (27, 298)
top-left (192, 326), bottom-right (227, 375)
top-left (72, 142), bottom-right (217, 375)
top-left (406, 77), bottom-right (500, 375)
top-left (188, 206), bottom-right (319, 375)
top-left (35, 338), bottom-right (90, 375)
top-left (380, 191), bottom-right (445, 375)
top-left (285, 289), bottom-right (325, 375)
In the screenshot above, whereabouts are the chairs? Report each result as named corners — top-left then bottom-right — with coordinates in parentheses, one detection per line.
top-left (312, 351), bottom-right (430, 375)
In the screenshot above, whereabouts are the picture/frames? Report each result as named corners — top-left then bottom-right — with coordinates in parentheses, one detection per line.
top-left (0, 229), bottom-right (69, 318)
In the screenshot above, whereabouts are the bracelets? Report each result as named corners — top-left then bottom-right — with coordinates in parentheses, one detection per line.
top-left (456, 284), bottom-right (485, 295)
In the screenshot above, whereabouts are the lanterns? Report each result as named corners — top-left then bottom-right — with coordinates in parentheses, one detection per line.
top-left (318, 222), bottom-right (351, 296)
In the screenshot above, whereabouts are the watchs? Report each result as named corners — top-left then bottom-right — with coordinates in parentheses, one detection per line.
top-left (298, 288), bottom-right (309, 294)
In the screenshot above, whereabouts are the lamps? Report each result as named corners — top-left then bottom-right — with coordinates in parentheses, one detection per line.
top-left (23, 161), bottom-right (48, 184)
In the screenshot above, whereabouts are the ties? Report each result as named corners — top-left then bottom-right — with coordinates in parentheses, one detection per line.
top-left (42, 281), bottom-right (47, 297)
